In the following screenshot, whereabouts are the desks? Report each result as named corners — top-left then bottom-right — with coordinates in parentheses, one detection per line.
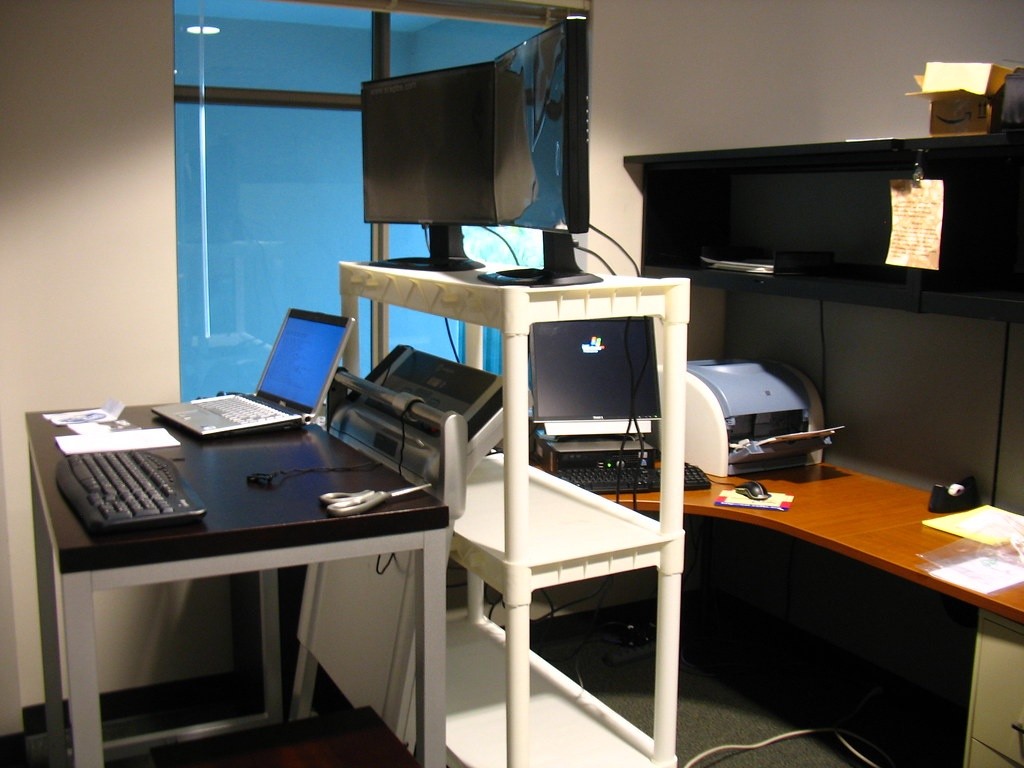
top-left (23, 406), bottom-right (449, 768)
top-left (530, 455), bottom-right (1024, 768)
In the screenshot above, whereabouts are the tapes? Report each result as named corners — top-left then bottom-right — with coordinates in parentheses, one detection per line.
top-left (948, 483), bottom-right (964, 495)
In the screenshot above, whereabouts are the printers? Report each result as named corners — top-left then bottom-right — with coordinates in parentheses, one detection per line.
top-left (685, 353), bottom-right (845, 477)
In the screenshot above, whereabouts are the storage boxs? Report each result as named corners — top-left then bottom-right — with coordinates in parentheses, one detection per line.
top-left (991, 58), bottom-right (1024, 135)
top-left (917, 62), bottom-right (1012, 135)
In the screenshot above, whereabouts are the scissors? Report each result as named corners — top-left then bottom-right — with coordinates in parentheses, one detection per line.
top-left (317, 480), bottom-right (435, 517)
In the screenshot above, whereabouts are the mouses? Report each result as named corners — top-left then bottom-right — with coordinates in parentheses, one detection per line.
top-left (735, 483), bottom-right (770, 500)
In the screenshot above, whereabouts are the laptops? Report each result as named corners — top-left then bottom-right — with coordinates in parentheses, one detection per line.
top-left (150, 308), bottom-right (356, 441)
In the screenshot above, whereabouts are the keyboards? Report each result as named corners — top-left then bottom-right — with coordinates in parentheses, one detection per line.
top-left (554, 462), bottom-right (712, 494)
top-left (56, 454), bottom-right (208, 538)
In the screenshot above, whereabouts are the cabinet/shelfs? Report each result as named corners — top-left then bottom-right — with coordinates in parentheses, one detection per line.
top-left (620, 138), bottom-right (1024, 322)
top-left (339, 257), bottom-right (691, 768)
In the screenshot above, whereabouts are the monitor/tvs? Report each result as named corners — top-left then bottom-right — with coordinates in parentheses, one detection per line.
top-left (528, 317), bottom-right (662, 451)
top-left (360, 58), bottom-right (497, 271)
top-left (479, 15), bottom-right (604, 286)
